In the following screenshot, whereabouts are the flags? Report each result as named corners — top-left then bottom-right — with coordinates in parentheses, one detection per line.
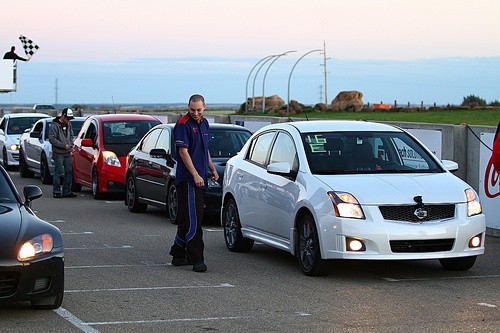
top-left (19, 34), bottom-right (39, 58)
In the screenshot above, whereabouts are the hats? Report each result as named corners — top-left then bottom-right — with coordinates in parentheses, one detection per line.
top-left (62, 108), bottom-right (75, 119)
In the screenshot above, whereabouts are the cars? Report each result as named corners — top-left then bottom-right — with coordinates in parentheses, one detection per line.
top-left (70, 114), bottom-right (163, 200)
top-left (34, 104), bottom-right (55, 109)
top-left (0, 164), bottom-right (64, 309)
top-left (126, 123), bottom-right (254, 224)
top-left (19, 117), bottom-right (86, 184)
top-left (221, 120), bottom-right (486, 276)
top-left (0, 113), bottom-right (52, 171)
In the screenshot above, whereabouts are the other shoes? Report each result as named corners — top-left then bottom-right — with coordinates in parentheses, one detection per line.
top-left (62, 193), bottom-right (77, 198)
top-left (171, 256), bottom-right (189, 266)
top-left (53, 193), bottom-right (62, 198)
top-left (192, 260), bottom-right (208, 272)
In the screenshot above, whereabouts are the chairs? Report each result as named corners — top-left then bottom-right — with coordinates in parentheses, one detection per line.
top-left (103, 126), bottom-right (112, 143)
top-left (283, 142), bottom-right (318, 172)
top-left (351, 143), bottom-right (376, 170)
top-left (134, 124), bottom-right (150, 139)
top-left (323, 138), bottom-right (347, 168)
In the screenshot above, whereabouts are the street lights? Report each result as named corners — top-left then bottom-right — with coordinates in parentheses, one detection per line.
top-left (247, 49), bottom-right (323, 115)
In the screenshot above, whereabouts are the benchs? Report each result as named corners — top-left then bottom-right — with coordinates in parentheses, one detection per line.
top-left (111, 132), bottom-right (135, 143)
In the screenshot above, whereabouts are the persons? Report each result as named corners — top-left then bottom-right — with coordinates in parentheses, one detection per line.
top-left (48, 108), bottom-right (78, 198)
top-left (168, 94), bottom-right (219, 271)
top-left (3, 46), bottom-right (28, 62)
